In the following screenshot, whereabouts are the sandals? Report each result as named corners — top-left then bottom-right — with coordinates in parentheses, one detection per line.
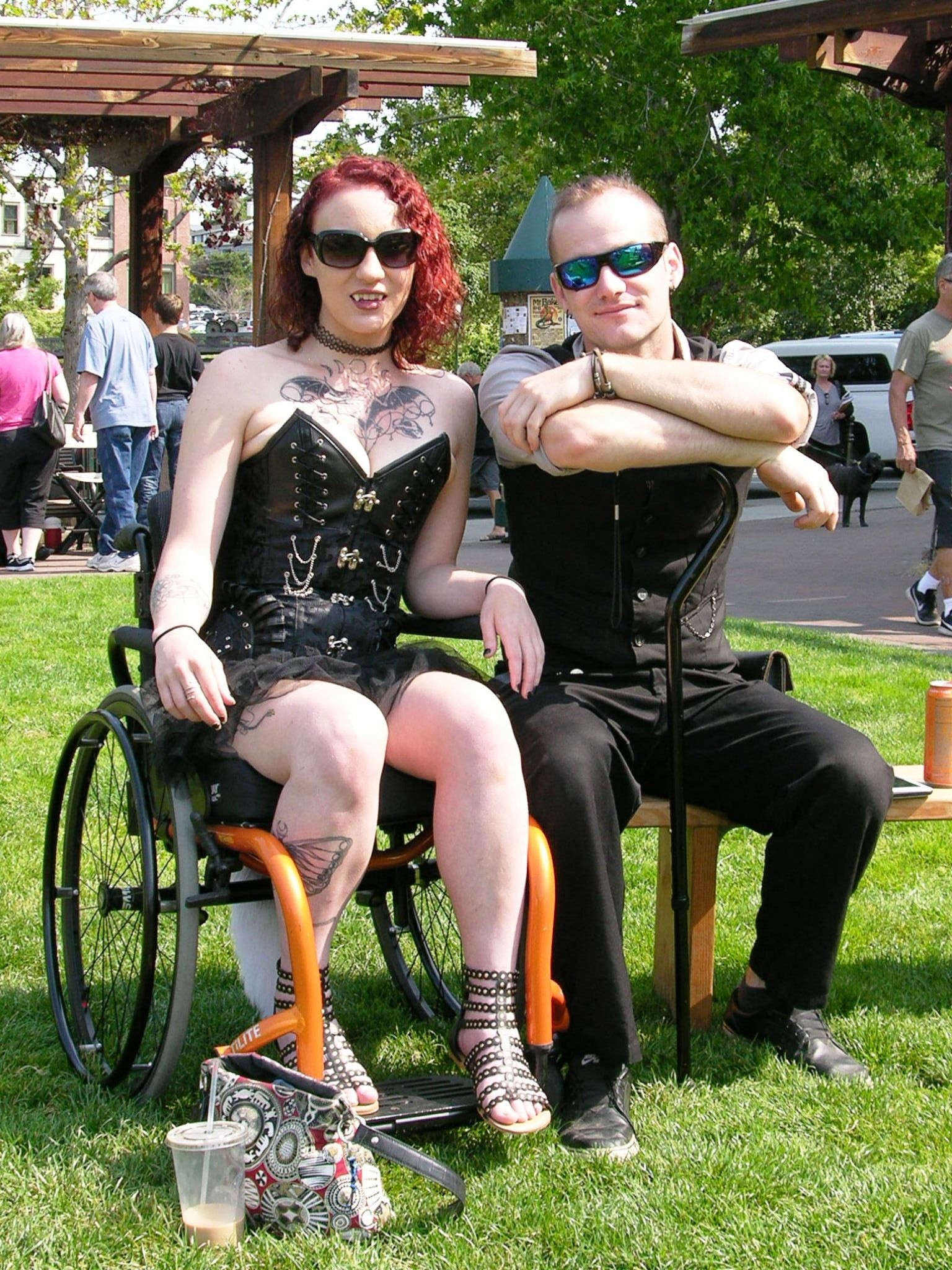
top-left (273, 958), bottom-right (377, 1113)
top-left (447, 961), bottom-right (551, 1133)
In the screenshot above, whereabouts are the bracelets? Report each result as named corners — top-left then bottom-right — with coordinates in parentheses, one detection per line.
top-left (152, 625), bottom-right (199, 648)
top-left (485, 576), bottom-right (526, 595)
top-left (580, 347), bottom-right (616, 400)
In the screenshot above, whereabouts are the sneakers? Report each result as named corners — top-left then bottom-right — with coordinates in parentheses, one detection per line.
top-left (86, 550), bottom-right (116, 570)
top-left (939, 609), bottom-right (952, 635)
top-left (558, 1055), bottom-right (640, 1158)
top-left (721, 989), bottom-right (865, 1083)
top-left (99, 553), bottom-right (141, 572)
top-left (906, 579), bottom-right (944, 627)
top-left (6, 552), bottom-right (35, 571)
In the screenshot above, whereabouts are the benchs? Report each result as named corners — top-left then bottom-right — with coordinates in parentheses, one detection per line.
top-left (628, 764), bottom-right (952, 1030)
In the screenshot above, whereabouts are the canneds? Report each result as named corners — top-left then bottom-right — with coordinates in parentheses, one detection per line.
top-left (923, 680), bottom-right (952, 789)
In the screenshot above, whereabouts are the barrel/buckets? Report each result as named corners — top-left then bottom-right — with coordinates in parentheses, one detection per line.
top-left (43, 517), bottom-right (62, 550)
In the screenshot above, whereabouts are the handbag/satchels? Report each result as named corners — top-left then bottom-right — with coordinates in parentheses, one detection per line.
top-left (30, 353), bottom-right (66, 448)
top-left (198, 1055), bottom-right (392, 1238)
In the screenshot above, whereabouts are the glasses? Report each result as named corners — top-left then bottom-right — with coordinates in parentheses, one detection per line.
top-left (307, 229), bottom-right (424, 270)
top-left (556, 241), bottom-right (672, 291)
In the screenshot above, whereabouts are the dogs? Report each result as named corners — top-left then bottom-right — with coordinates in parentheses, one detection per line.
top-left (820, 450), bottom-right (889, 530)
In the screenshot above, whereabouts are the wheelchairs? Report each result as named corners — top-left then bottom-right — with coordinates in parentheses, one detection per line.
top-left (41, 486), bottom-right (571, 1142)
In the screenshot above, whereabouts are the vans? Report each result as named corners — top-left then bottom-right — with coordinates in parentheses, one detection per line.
top-left (756, 330), bottom-right (918, 462)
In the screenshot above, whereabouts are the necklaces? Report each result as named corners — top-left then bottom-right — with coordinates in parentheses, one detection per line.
top-left (313, 321), bottom-right (395, 356)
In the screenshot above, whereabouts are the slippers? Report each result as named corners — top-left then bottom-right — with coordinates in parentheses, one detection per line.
top-left (479, 533), bottom-right (506, 542)
top-left (500, 536), bottom-right (509, 543)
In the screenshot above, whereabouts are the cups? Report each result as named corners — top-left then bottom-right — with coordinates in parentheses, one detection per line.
top-left (165, 1121), bottom-right (248, 1249)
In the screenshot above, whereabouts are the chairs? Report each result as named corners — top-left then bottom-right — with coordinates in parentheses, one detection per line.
top-left (51, 424), bottom-right (105, 555)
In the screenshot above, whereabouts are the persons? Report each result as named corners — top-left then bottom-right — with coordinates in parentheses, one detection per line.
top-left (72, 271), bottom-right (159, 572)
top-left (0, 312), bottom-right (71, 572)
top-left (132, 293), bottom-right (205, 529)
top-left (803, 354), bottom-right (854, 526)
top-left (150, 155), bottom-right (554, 1136)
top-left (456, 362), bottom-right (506, 541)
top-left (888, 253), bottom-right (952, 637)
top-left (479, 169), bottom-right (892, 1163)
